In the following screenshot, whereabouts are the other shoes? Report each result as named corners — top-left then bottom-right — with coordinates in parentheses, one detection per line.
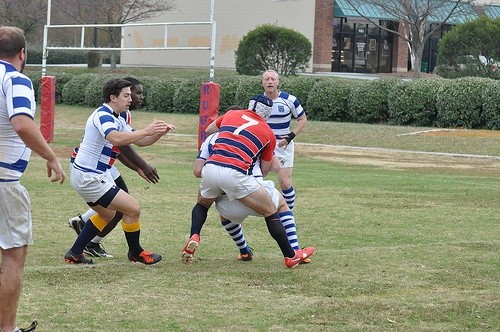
top-left (15, 320), bottom-right (39, 332)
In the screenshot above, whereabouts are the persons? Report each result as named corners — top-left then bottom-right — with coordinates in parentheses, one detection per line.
top-left (180, 69), bottom-right (316, 267)
top-left (64, 77), bottom-right (159, 259)
top-left (0, 26), bottom-right (66, 332)
top-left (63, 80), bottom-right (163, 266)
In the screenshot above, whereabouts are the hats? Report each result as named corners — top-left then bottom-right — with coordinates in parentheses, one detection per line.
top-left (247, 93), bottom-right (274, 121)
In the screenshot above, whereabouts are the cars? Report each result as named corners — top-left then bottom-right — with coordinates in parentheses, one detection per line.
top-left (333, 48), bottom-right (372, 73)
top-left (442, 54), bottom-right (486, 71)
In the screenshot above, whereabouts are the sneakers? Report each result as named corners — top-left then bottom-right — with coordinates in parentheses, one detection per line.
top-left (297, 247), bottom-right (312, 263)
top-left (83, 241), bottom-right (114, 259)
top-left (179, 234), bottom-right (200, 265)
top-left (236, 247), bottom-right (256, 261)
top-left (127, 250), bottom-right (162, 265)
top-left (63, 248), bottom-right (93, 265)
top-left (67, 212), bottom-right (86, 235)
top-left (283, 246), bottom-right (316, 268)
